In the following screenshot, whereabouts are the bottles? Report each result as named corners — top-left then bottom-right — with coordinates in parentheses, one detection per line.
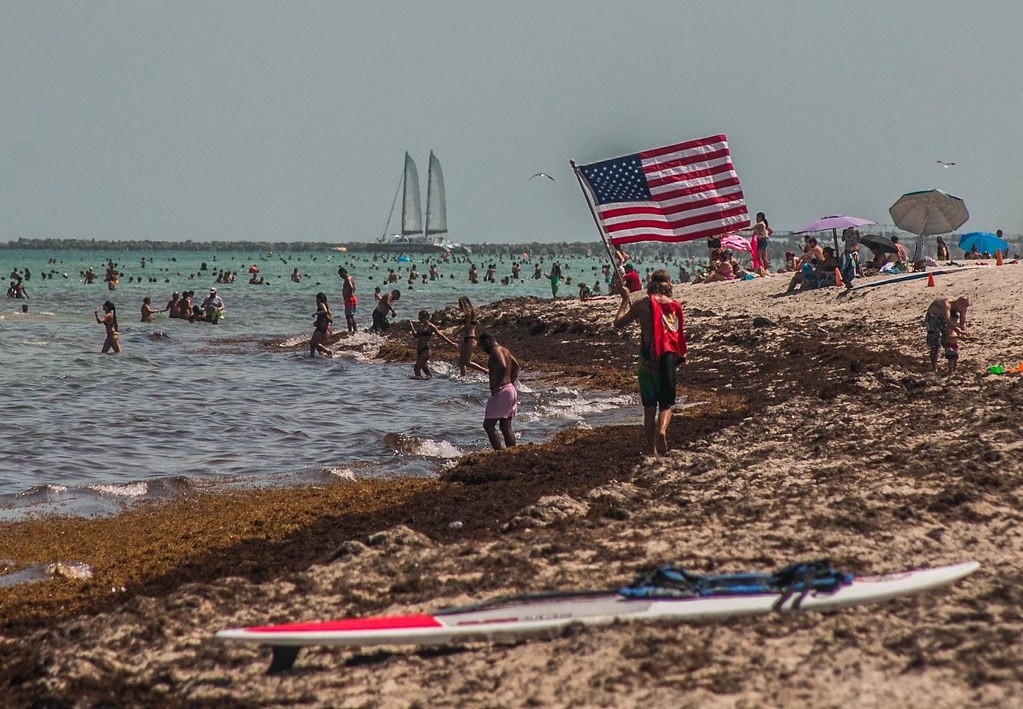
top-left (987, 366), bottom-right (1002, 374)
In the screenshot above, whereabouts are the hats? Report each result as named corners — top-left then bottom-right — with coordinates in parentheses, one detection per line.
top-left (89, 267), bottom-right (94, 271)
top-left (725, 248), bottom-right (732, 254)
top-left (209, 287), bottom-right (218, 294)
top-left (623, 264), bottom-right (633, 269)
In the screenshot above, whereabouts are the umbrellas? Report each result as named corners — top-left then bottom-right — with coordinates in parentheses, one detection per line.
top-left (889, 188), bottom-right (971, 239)
top-left (792, 214), bottom-right (877, 257)
top-left (958, 231), bottom-right (1009, 254)
top-left (858, 233), bottom-right (898, 253)
top-left (719, 236), bottom-right (753, 252)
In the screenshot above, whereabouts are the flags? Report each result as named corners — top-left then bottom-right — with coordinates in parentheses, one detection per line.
top-left (578, 134), bottom-right (751, 246)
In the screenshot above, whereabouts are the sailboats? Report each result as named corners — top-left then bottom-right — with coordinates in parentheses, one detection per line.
top-left (352, 150), bottom-right (472, 256)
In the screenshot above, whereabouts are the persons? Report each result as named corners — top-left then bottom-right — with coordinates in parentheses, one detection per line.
top-left (84, 258), bottom-right (119, 289)
top-left (215, 271), bottom-right (265, 284)
top-left (95, 301), bottom-right (122, 353)
top-left (501, 250), bottom-right (612, 299)
top-left (923, 293), bottom-right (972, 370)
top-left (738, 212), bottom-right (908, 293)
top-left (614, 269), bottom-right (687, 457)
top-left (371, 289), bottom-right (401, 329)
top-left (453, 296), bottom-right (489, 376)
top-left (337, 267), bottom-right (357, 331)
top-left (613, 244), bottom-right (629, 276)
top-left (6, 268), bottom-right (30, 300)
top-left (140, 287), bottom-right (225, 324)
top-left (479, 332), bottom-right (520, 450)
top-left (291, 268), bottom-right (301, 283)
top-left (388, 264), bottom-right (437, 284)
top-left (468, 263), bottom-right (496, 283)
top-left (622, 234), bottom-right (742, 293)
top-left (935, 236), bottom-right (990, 260)
top-left (406, 310), bottom-right (458, 377)
top-left (309, 291), bottom-right (334, 356)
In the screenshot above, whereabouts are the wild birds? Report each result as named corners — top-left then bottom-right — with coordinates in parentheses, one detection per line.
top-left (528, 172), bottom-right (556, 183)
top-left (936, 161), bottom-right (956, 168)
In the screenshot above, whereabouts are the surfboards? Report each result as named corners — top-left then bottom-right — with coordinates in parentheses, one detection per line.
top-left (211, 559), bottom-right (979, 647)
